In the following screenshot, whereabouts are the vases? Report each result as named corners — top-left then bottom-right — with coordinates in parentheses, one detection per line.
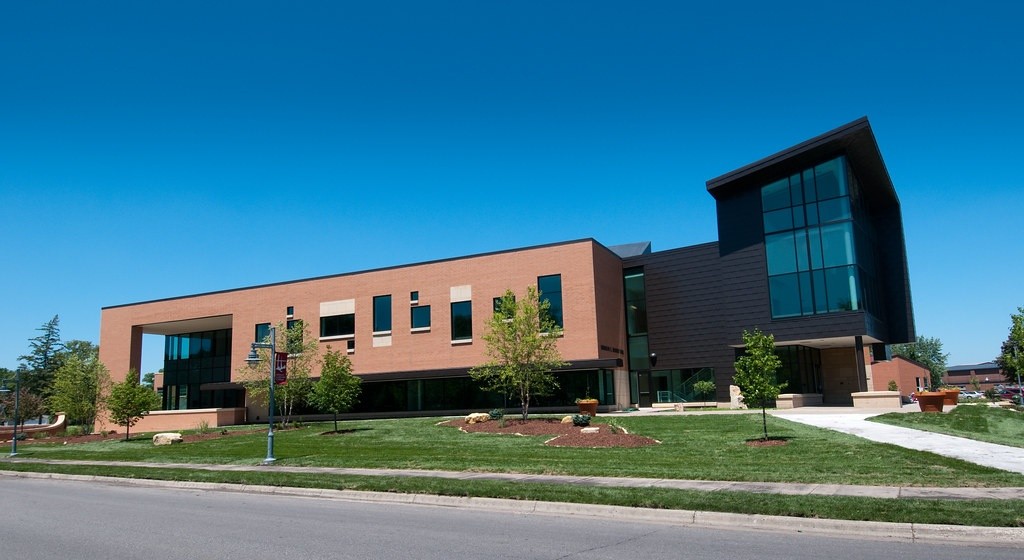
top-left (940, 390), bottom-right (960, 405)
top-left (915, 392), bottom-right (946, 412)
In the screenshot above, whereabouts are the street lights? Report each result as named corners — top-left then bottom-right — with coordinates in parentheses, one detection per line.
top-left (0, 369), bottom-right (21, 456)
top-left (244, 327), bottom-right (278, 463)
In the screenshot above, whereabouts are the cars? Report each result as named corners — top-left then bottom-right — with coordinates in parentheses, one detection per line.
top-left (987, 387), bottom-right (1024, 401)
top-left (957, 391), bottom-right (983, 403)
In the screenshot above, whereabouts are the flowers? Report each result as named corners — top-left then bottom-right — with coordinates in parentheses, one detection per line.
top-left (938, 383), bottom-right (961, 390)
top-left (920, 385), bottom-right (942, 392)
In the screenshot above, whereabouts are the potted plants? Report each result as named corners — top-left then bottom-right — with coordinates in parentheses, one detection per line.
top-left (575, 391), bottom-right (598, 417)
